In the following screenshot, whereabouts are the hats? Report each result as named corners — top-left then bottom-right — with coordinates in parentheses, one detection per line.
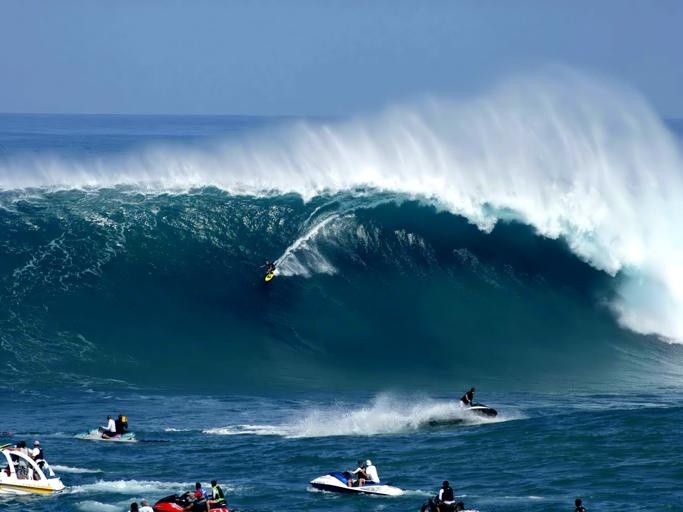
top-left (366, 460), bottom-right (371, 465)
top-left (34, 441), bottom-right (39, 444)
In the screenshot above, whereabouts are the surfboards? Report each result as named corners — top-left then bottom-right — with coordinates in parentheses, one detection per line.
top-left (265, 271), bottom-right (274, 281)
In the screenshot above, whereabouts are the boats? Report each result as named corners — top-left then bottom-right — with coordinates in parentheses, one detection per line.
top-left (152, 490), bottom-right (228, 512)
top-left (420, 496), bottom-right (463, 512)
top-left (264, 269), bottom-right (277, 282)
top-left (428, 402), bottom-right (497, 427)
top-left (87, 427), bottom-right (135, 442)
top-left (0, 442), bottom-right (64, 495)
top-left (308, 471), bottom-right (403, 496)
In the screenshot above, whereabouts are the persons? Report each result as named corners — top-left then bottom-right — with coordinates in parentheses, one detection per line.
top-left (184, 482), bottom-right (208, 512)
top-left (358, 460), bottom-right (380, 487)
top-left (438, 480), bottom-right (456, 512)
top-left (346, 460), bottom-right (366, 487)
top-left (3, 440), bottom-right (44, 481)
top-left (100, 415), bottom-right (116, 439)
top-left (115, 415), bottom-right (129, 434)
top-left (573, 499), bottom-right (588, 512)
top-left (459, 388), bottom-right (476, 409)
top-left (128, 500), bottom-right (154, 512)
top-left (265, 261), bottom-right (276, 276)
top-left (206, 479), bottom-right (224, 512)
